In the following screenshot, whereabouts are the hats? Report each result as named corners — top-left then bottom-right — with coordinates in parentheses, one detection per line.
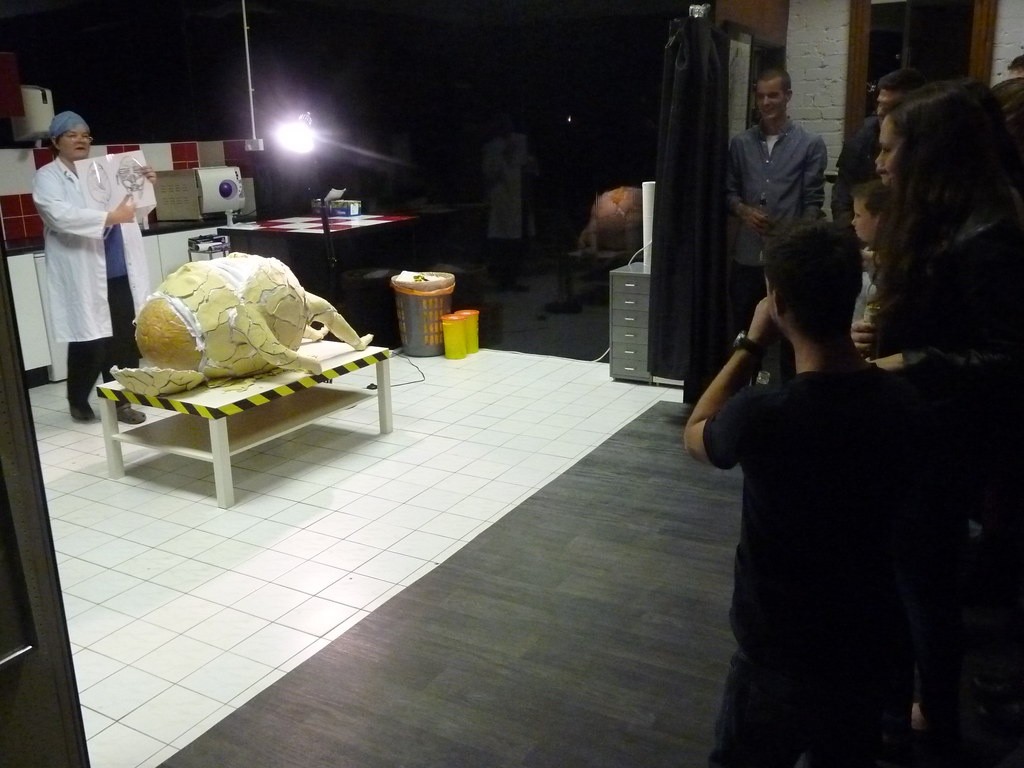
top-left (48, 110), bottom-right (91, 138)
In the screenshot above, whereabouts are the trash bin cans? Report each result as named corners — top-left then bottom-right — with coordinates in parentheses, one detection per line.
top-left (455, 309), bottom-right (480, 354)
top-left (441, 313), bottom-right (467, 360)
top-left (338, 267), bottom-right (397, 347)
top-left (391, 271), bottom-right (455, 357)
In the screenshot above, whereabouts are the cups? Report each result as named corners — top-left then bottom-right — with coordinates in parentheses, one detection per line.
top-left (455, 310), bottom-right (480, 354)
top-left (442, 314), bottom-right (465, 360)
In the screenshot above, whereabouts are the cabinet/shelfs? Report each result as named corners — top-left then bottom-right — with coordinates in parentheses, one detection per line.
top-left (609, 262), bottom-right (653, 385)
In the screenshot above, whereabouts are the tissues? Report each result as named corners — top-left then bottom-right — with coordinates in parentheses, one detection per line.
top-left (311, 188), bottom-right (361, 217)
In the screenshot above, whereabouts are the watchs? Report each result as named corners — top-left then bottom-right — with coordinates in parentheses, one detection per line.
top-left (732, 331), bottom-right (767, 358)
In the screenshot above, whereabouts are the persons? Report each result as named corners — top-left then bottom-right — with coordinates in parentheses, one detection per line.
top-left (478, 104), bottom-right (537, 293)
top-left (32, 112), bottom-right (158, 424)
top-left (684, 57), bottom-right (1024, 768)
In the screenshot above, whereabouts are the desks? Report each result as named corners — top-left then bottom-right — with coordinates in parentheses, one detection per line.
top-left (96, 338), bottom-right (394, 510)
top-left (217, 216), bottom-right (421, 303)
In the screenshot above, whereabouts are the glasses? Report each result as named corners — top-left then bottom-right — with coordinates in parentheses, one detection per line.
top-left (61, 135), bottom-right (93, 145)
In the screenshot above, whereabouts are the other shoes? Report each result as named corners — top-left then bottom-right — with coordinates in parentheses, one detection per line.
top-left (975, 696), bottom-right (1024, 739)
top-left (116, 402), bottom-right (146, 424)
top-left (913, 688), bottom-right (964, 757)
top-left (70, 401), bottom-right (95, 421)
top-left (973, 664), bottom-right (1024, 703)
